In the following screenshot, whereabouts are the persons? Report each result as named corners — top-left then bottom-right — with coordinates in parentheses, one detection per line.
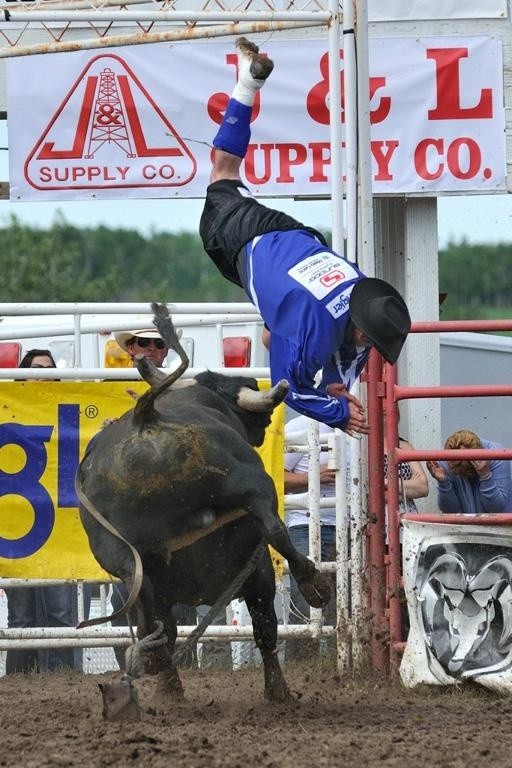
top-left (194, 29), bottom-right (414, 440)
top-left (276, 412), bottom-right (351, 664)
top-left (424, 427), bottom-right (512, 513)
top-left (101, 326), bottom-right (184, 670)
top-left (2, 347), bottom-right (80, 674)
top-left (380, 398), bottom-right (432, 661)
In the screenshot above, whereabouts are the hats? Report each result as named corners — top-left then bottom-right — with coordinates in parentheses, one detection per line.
top-left (112, 328), bottom-right (183, 354)
top-left (348, 277), bottom-right (412, 367)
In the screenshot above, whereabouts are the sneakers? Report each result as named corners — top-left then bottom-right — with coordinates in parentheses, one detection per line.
top-left (234, 36), bottom-right (275, 90)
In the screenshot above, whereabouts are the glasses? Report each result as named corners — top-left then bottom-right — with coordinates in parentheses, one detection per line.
top-left (126, 338), bottom-right (168, 349)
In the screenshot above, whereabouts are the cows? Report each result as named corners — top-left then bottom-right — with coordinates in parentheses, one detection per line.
top-left (70, 297), bottom-right (333, 713)
